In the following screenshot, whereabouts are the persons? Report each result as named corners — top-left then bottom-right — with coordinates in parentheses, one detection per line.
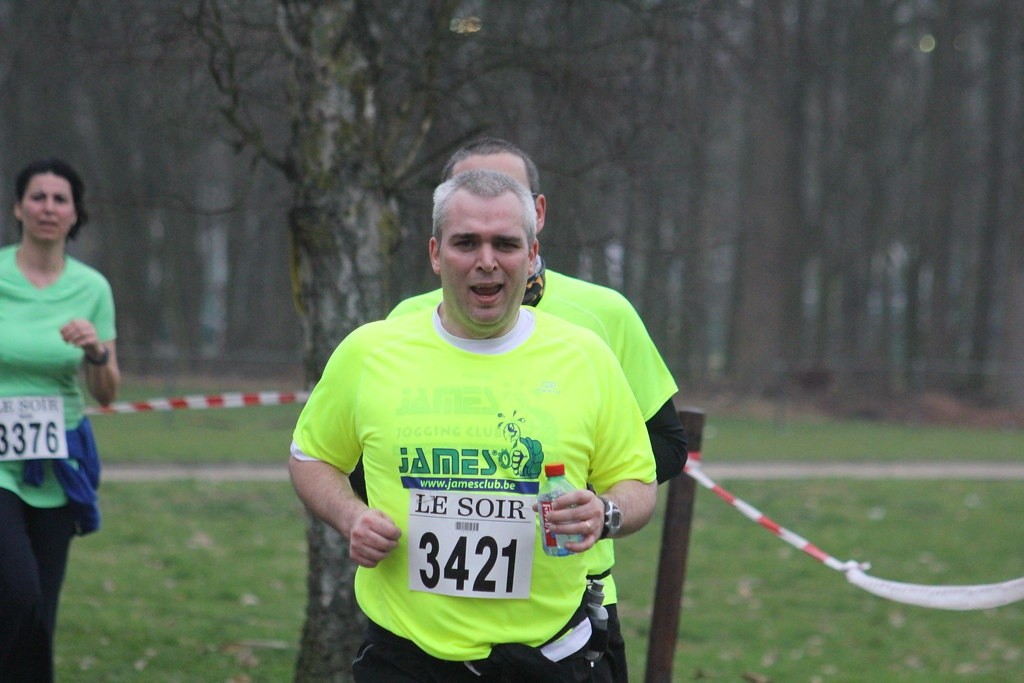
top-left (286, 171), bottom-right (658, 682)
top-left (0, 160), bottom-right (122, 682)
top-left (442, 136), bottom-right (688, 483)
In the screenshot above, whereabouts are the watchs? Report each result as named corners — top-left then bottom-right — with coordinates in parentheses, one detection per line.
top-left (596, 493), bottom-right (623, 539)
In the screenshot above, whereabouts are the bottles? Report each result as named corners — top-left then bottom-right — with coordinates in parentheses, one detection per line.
top-left (539, 462), bottom-right (583, 557)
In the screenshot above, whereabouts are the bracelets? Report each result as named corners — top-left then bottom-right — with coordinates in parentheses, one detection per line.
top-left (84, 345), bottom-right (112, 365)
